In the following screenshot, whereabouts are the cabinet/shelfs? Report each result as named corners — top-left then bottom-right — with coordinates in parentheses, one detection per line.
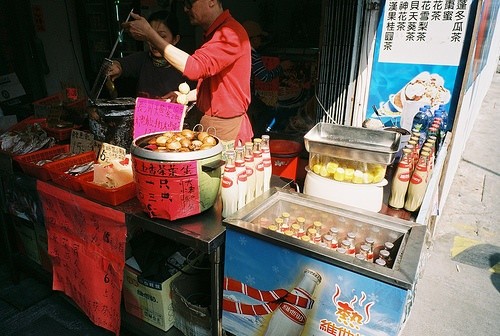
top-left (0, 168), bottom-right (296, 336)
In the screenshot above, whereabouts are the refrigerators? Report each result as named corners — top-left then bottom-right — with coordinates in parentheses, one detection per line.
top-left (229, 185), bottom-right (427, 336)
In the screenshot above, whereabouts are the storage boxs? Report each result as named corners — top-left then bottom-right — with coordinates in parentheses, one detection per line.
top-left (12, 216), bottom-right (41, 266)
top-left (170, 254), bottom-right (213, 336)
top-left (123, 231), bottom-right (207, 333)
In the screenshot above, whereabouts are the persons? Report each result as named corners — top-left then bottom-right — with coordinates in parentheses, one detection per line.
top-left (105, 10), bottom-right (199, 130)
top-left (119, 0), bottom-right (253, 148)
top-left (240, 19), bottom-right (295, 138)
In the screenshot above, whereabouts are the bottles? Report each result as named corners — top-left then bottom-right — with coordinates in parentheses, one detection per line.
top-left (389, 105), bottom-right (449, 207)
top-left (223, 135), bottom-right (273, 218)
top-left (264, 269), bottom-right (322, 336)
top-left (270, 211), bottom-right (394, 267)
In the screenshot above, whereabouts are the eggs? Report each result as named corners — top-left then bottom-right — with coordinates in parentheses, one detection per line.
top-left (156, 127), bottom-right (217, 152)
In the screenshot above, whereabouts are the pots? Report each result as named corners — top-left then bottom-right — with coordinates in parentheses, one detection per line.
top-left (132, 124), bottom-right (226, 217)
top-left (302, 165), bottom-right (389, 212)
